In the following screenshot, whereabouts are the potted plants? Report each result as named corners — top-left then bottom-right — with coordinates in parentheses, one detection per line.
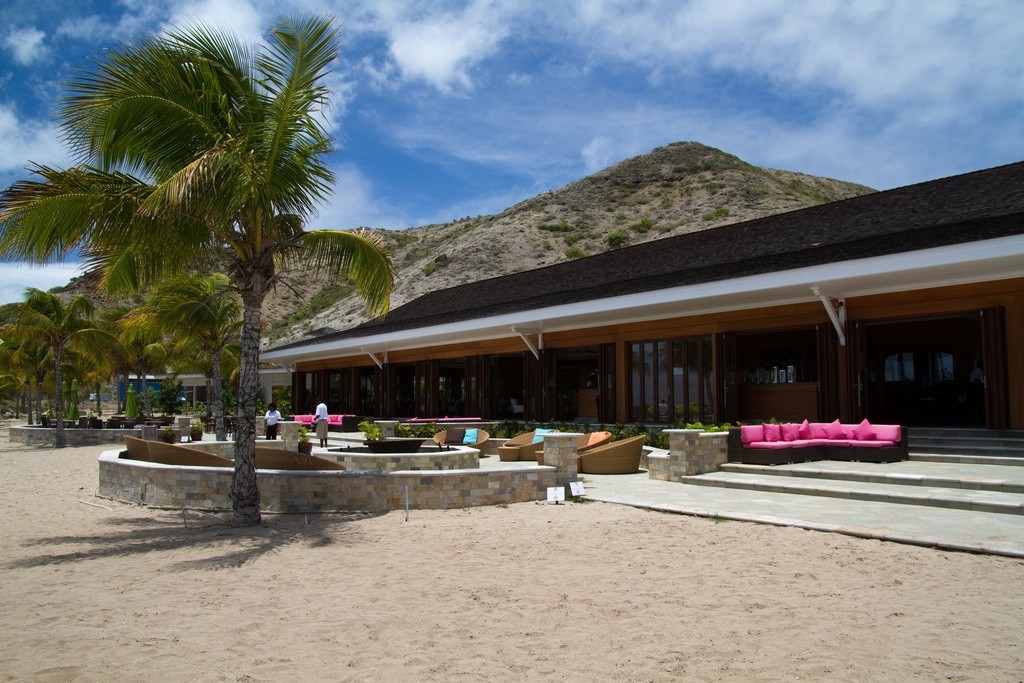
top-left (298, 426), bottom-right (312, 455)
top-left (189, 421), bottom-right (206, 441)
top-left (161, 427), bottom-right (175, 443)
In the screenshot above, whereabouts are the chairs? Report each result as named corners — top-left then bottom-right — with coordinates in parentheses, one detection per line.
top-left (41, 414), bottom-right (236, 437)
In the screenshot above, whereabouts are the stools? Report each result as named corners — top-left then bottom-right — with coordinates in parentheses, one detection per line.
top-left (497, 447), bottom-right (520, 462)
top-left (534, 450), bottom-right (544, 465)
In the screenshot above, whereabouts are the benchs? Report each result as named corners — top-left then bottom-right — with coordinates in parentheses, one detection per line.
top-left (123, 435), bottom-right (234, 467)
top-left (576, 431), bottom-right (611, 450)
top-left (504, 429), bottom-right (560, 461)
top-left (255, 446), bottom-right (344, 472)
top-left (433, 426), bottom-right (490, 456)
top-left (582, 434), bottom-right (645, 475)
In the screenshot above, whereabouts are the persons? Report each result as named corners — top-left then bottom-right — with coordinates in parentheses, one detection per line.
top-left (264, 402), bottom-right (282, 441)
top-left (314, 395), bottom-right (329, 448)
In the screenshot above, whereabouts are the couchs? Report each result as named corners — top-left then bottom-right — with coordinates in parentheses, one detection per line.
top-left (728, 424), bottom-right (909, 464)
top-left (285, 414), bottom-right (363, 433)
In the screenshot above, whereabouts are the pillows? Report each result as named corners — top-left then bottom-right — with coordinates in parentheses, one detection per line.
top-left (588, 432), bottom-right (609, 445)
top-left (780, 422), bottom-right (796, 441)
top-left (763, 422), bottom-right (778, 442)
top-left (443, 427), bottom-right (466, 443)
top-left (821, 419), bottom-right (847, 439)
top-left (798, 419), bottom-right (812, 439)
top-left (532, 428), bottom-right (553, 443)
top-left (852, 418), bottom-right (879, 441)
top-left (464, 428), bottom-right (478, 444)
